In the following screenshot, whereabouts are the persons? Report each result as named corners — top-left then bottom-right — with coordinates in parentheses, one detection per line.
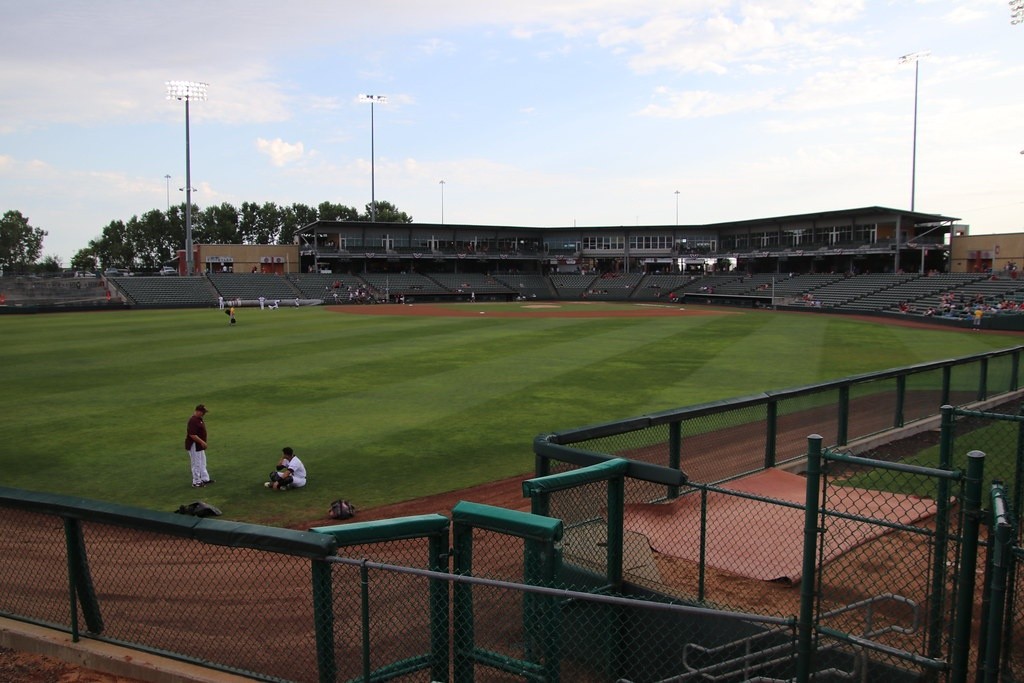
top-left (268, 300), bottom-right (282, 310)
top-left (895, 259), bottom-right (1024, 332)
top-left (229, 306), bottom-right (236, 324)
top-left (669, 283), bottom-right (823, 308)
top-left (456, 282), bottom-right (476, 303)
top-left (184, 404), bottom-right (216, 488)
top-left (264, 447), bottom-right (306, 490)
top-left (333, 278), bottom-right (404, 305)
top-left (582, 264), bottom-right (607, 298)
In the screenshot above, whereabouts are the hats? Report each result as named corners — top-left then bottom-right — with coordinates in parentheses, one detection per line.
top-left (196, 404), bottom-right (208, 413)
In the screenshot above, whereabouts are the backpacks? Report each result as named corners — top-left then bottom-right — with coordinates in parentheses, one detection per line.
top-left (331, 500), bottom-right (355, 519)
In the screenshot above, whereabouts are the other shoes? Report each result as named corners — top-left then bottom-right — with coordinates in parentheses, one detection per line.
top-left (202, 479), bottom-right (216, 483)
top-left (265, 482), bottom-right (274, 487)
top-left (279, 485), bottom-right (287, 490)
top-left (192, 483), bottom-right (207, 488)
